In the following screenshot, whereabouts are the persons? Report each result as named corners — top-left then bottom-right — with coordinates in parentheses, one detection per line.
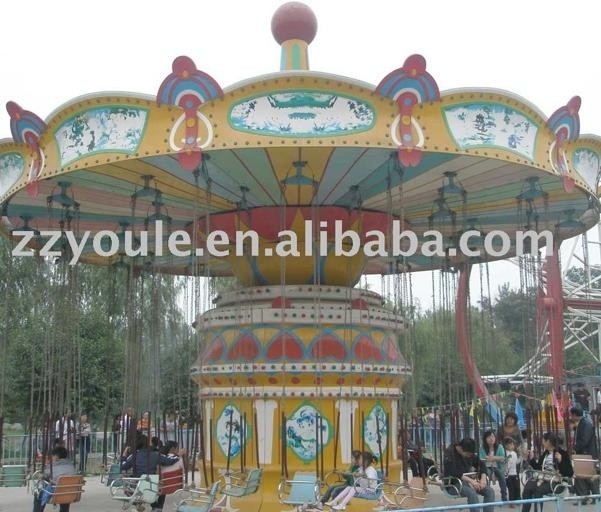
top-left (442, 438), bottom-right (496, 512)
top-left (575, 382), bottom-right (592, 417)
top-left (400, 427), bottom-right (439, 480)
top-left (325, 451), bottom-right (380, 511)
top-left (26, 404), bottom-right (199, 512)
top-left (595, 385), bottom-right (600, 424)
top-left (480, 404), bottom-right (601, 512)
top-left (396, 435), bottom-right (425, 479)
top-left (308, 449), bottom-right (362, 511)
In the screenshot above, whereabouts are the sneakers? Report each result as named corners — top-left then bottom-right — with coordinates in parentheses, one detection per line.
top-left (325, 500), bottom-right (338, 506)
top-left (332, 504), bottom-right (345, 510)
